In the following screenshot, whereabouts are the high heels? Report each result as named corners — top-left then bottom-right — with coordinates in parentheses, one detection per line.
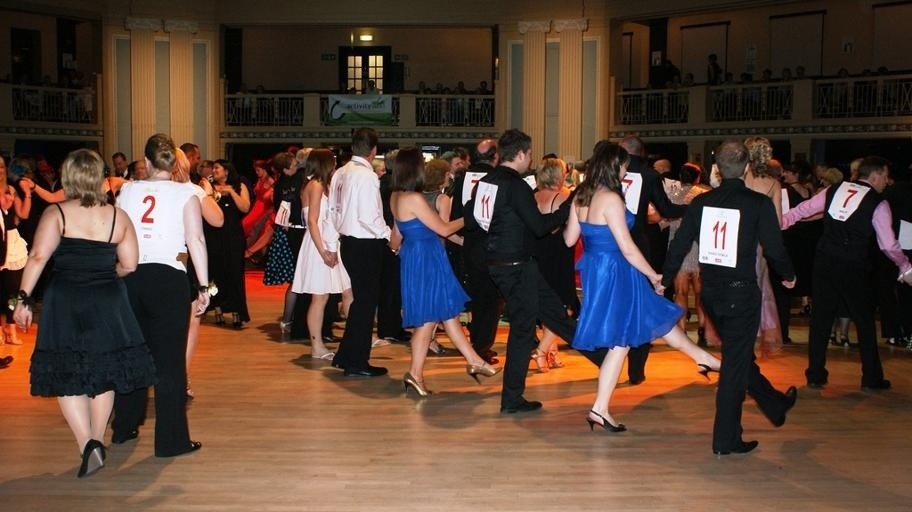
top-left (581, 409), bottom-right (630, 433)
top-left (1, 321), bottom-right (24, 346)
top-left (280, 320), bottom-right (292, 334)
top-left (547, 349), bottom-right (565, 367)
top-left (528, 346), bottom-right (550, 375)
top-left (694, 356), bottom-right (723, 381)
top-left (839, 332), bottom-right (849, 346)
top-left (830, 332), bottom-right (837, 345)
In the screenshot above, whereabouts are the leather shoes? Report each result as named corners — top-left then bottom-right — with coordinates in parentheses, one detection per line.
top-left (76, 428), bottom-right (201, 477)
top-left (859, 374), bottom-right (892, 391)
top-left (500, 399), bottom-right (542, 415)
top-left (403, 339), bottom-right (501, 398)
top-left (324, 330), bottom-right (412, 378)
top-left (715, 367), bottom-right (827, 456)
top-left (888, 337), bottom-right (907, 347)
top-left (2, 354), bottom-right (13, 368)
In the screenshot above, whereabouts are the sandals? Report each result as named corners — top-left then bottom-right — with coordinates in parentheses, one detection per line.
top-left (231, 313), bottom-right (243, 330)
top-left (216, 312), bottom-right (225, 325)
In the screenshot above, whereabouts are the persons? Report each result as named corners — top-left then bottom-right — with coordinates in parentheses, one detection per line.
top-left (1, 134), bottom-right (251, 476)
top-left (234, 83), bottom-right (273, 121)
top-left (647, 56), bottom-right (895, 121)
top-left (53, 68), bottom-right (95, 120)
top-left (241, 128), bottom-right (911, 454)
top-left (364, 79), bottom-right (493, 126)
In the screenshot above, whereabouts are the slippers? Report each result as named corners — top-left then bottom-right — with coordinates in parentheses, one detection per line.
top-left (310, 351), bottom-right (336, 363)
